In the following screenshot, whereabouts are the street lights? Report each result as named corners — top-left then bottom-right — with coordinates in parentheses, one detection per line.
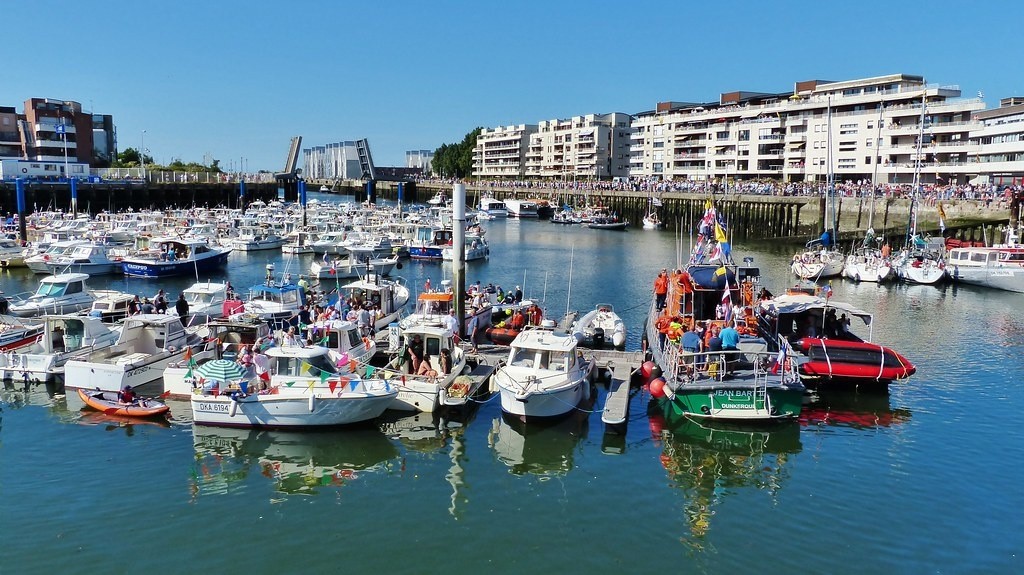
top-left (141, 130), bottom-right (146, 179)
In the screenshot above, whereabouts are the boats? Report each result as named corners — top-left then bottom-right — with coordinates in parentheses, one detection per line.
top-left (0, 87), bottom-right (1024, 424)
top-left (77, 388), bottom-right (171, 418)
top-left (188, 340), bottom-right (404, 431)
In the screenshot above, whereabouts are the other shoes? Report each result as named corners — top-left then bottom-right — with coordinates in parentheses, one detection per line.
top-left (468, 349), bottom-right (474, 354)
top-left (472, 352), bottom-right (478, 355)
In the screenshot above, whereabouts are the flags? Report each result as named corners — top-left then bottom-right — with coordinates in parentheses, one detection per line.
top-left (722, 282), bottom-right (736, 322)
top-left (55, 124), bottom-right (65, 132)
top-left (687, 196), bottom-right (735, 266)
top-left (907, 90), bottom-right (945, 237)
top-left (652, 196), bottom-right (663, 207)
top-left (772, 347), bottom-right (786, 375)
top-left (827, 283), bottom-right (833, 300)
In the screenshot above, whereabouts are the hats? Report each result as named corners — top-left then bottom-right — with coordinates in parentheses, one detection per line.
top-left (178, 292), bottom-right (184, 298)
top-left (298, 274), bottom-right (305, 279)
top-left (516, 285), bottom-right (520, 290)
top-left (534, 305), bottom-right (538, 308)
top-left (508, 290), bottom-right (512, 293)
top-left (517, 308), bottom-right (521, 311)
top-left (124, 385), bottom-right (133, 391)
top-left (365, 302), bottom-right (373, 307)
top-left (141, 297), bottom-right (148, 302)
top-left (242, 354), bottom-right (252, 363)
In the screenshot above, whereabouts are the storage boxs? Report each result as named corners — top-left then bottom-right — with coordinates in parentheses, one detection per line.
top-left (449, 380), bottom-right (473, 397)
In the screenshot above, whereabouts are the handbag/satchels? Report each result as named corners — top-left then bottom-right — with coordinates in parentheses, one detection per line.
top-left (453, 331), bottom-right (459, 343)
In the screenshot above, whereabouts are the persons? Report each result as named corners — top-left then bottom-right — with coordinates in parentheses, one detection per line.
top-left (404, 175), bottom-right (1024, 226)
top-left (881, 244), bottom-right (891, 267)
top-left (119, 385), bottom-right (144, 405)
top-left (127, 269), bottom-right (851, 388)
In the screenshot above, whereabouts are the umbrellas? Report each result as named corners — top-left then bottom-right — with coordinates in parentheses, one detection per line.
top-left (196, 360), bottom-right (248, 381)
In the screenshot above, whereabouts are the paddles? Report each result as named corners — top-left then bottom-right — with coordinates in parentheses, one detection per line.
top-left (130, 389), bottom-right (172, 406)
